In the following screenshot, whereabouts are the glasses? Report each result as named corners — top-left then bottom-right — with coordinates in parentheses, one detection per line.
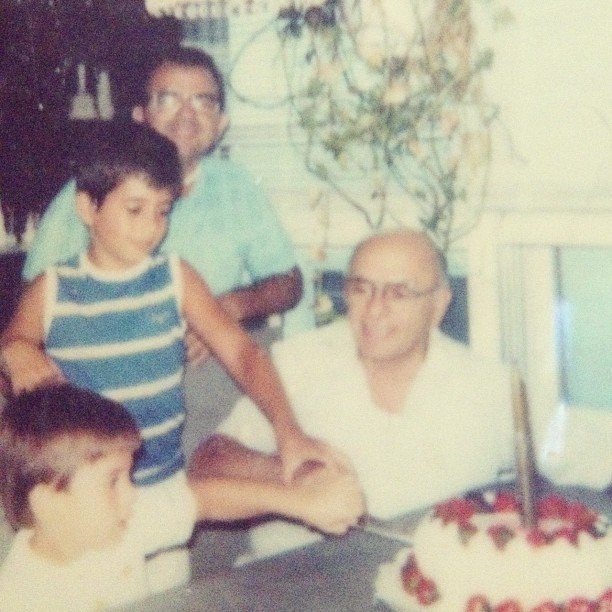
top-left (345, 279), bottom-right (436, 305)
top-left (151, 93), bottom-right (218, 110)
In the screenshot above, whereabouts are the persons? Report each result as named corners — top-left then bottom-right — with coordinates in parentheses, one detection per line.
top-left (0, 385), bottom-right (367, 610)
top-left (19, 46), bottom-right (304, 368)
top-left (1, 125), bottom-right (359, 493)
top-left (188, 230), bottom-right (529, 575)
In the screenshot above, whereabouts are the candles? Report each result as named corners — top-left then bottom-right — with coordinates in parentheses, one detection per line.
top-left (511, 373), bottom-right (534, 532)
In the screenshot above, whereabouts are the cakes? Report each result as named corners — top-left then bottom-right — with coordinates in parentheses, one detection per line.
top-left (369, 487), bottom-right (612, 611)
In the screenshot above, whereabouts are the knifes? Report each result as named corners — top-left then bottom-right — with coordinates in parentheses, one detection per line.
top-left (341, 519), bottom-right (429, 547)
